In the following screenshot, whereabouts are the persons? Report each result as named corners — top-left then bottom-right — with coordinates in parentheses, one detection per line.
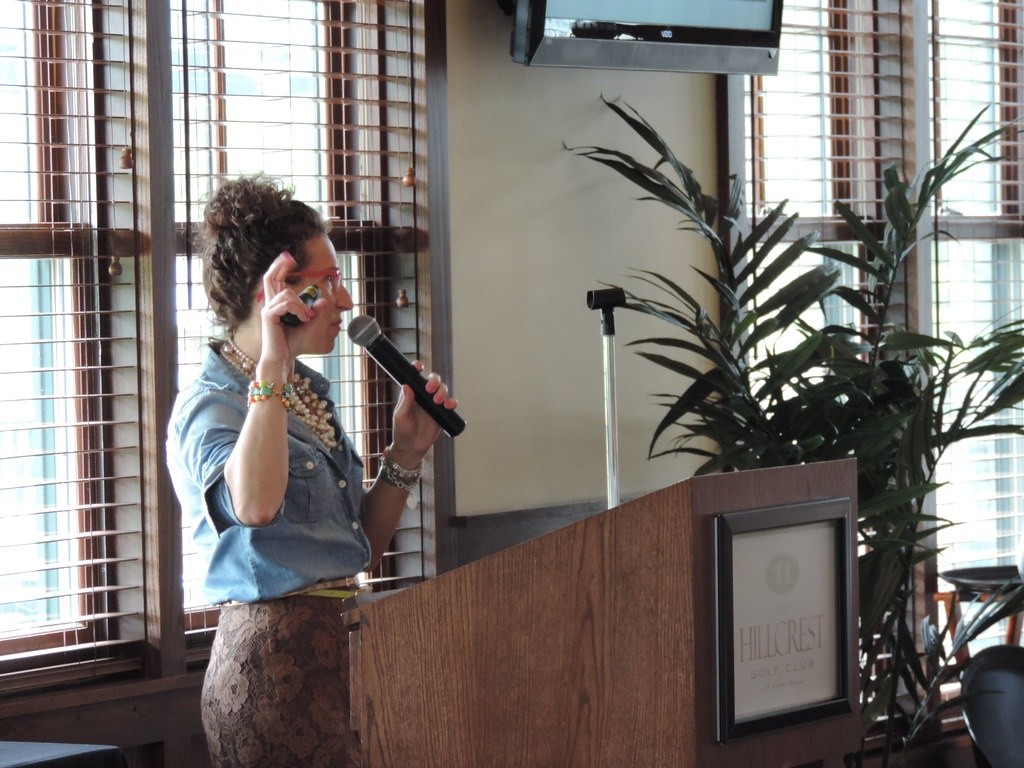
top-left (164, 174), bottom-right (459, 767)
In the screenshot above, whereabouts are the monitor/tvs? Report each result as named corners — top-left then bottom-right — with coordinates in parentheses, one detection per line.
top-left (510, 0), bottom-right (783, 76)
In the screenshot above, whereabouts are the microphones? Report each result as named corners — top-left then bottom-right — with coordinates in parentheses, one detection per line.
top-left (348, 315), bottom-right (466, 438)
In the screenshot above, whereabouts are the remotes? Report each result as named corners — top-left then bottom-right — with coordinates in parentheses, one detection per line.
top-left (279, 284), bottom-right (322, 328)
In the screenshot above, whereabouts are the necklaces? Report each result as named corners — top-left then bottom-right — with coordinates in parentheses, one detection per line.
top-left (220, 335), bottom-right (339, 456)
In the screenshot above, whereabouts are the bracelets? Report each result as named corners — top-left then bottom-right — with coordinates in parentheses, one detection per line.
top-left (379, 449), bottom-right (424, 510)
top-left (247, 380), bottom-right (294, 412)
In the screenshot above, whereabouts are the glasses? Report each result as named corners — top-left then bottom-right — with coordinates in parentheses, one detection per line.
top-left (255, 265), bottom-right (341, 304)
